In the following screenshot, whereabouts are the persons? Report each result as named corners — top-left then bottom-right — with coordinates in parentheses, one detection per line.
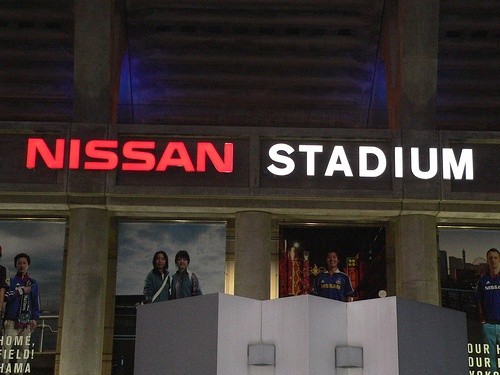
top-left (474, 248), bottom-right (500, 375)
top-left (143, 250), bottom-right (202, 304)
top-left (0, 246), bottom-right (41, 375)
top-left (311, 248), bottom-right (357, 302)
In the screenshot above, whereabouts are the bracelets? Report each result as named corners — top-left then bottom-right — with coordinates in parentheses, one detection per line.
top-left (481, 321), bottom-right (485, 324)
